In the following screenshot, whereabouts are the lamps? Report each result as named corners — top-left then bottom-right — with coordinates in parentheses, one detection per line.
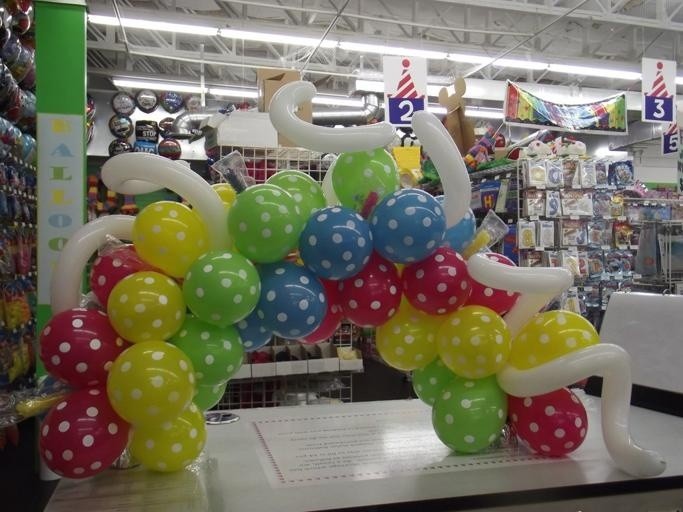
top-left (88, 12), bottom-right (220, 38)
top-left (425, 104), bottom-right (507, 122)
top-left (110, 75), bottom-right (207, 100)
top-left (448, 52), bottom-right (547, 72)
top-left (211, 86), bottom-right (363, 110)
top-left (339, 39), bottom-right (446, 65)
top-left (550, 63), bottom-right (683, 86)
top-left (219, 25), bottom-right (338, 52)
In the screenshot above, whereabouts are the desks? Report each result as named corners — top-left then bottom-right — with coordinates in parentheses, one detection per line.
top-left (45, 388), bottom-right (682, 512)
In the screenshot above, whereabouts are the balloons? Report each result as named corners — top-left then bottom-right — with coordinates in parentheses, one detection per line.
top-left (185, 375), bottom-right (246, 419)
top-left (403, 246), bottom-right (472, 310)
top-left (505, 341), bottom-right (673, 474)
top-left (110, 271), bottom-right (186, 343)
top-left (505, 310), bottom-right (605, 377)
top-left (404, 346), bottom-right (468, 402)
top-left (48, 212), bottom-right (138, 307)
top-left (269, 75), bottom-right (399, 158)
top-left (227, 185), bottom-right (299, 270)
top-left (423, 373), bottom-right (507, 449)
top-left (231, 293), bottom-right (270, 357)
top-left (376, 313), bottom-right (441, 369)
top-left (257, 272), bottom-right (275, 290)
top-left (428, 307), bottom-right (508, 376)
top-left (510, 392), bottom-right (593, 459)
top-left (435, 191), bottom-right (486, 259)
top-left (41, 390), bottom-right (128, 482)
top-left (42, 305), bottom-right (114, 387)
top-left (183, 254), bottom-right (261, 325)
top-left (367, 186), bottom-right (447, 262)
top-left (132, 200), bottom-right (215, 275)
top-left (95, 151), bottom-right (231, 244)
top-left (299, 206), bottom-right (369, 281)
top-left (403, 102), bottom-right (480, 238)
top-left (106, 345), bottom-right (202, 429)
top-left (252, 263), bottom-right (332, 340)
top-left (466, 247), bottom-right (575, 318)
top-left (264, 165), bottom-right (329, 235)
top-left (172, 323), bottom-right (243, 389)
top-left (343, 258), bottom-right (407, 331)
top-left (124, 404), bottom-right (211, 471)
top-left (328, 145), bottom-right (403, 205)
